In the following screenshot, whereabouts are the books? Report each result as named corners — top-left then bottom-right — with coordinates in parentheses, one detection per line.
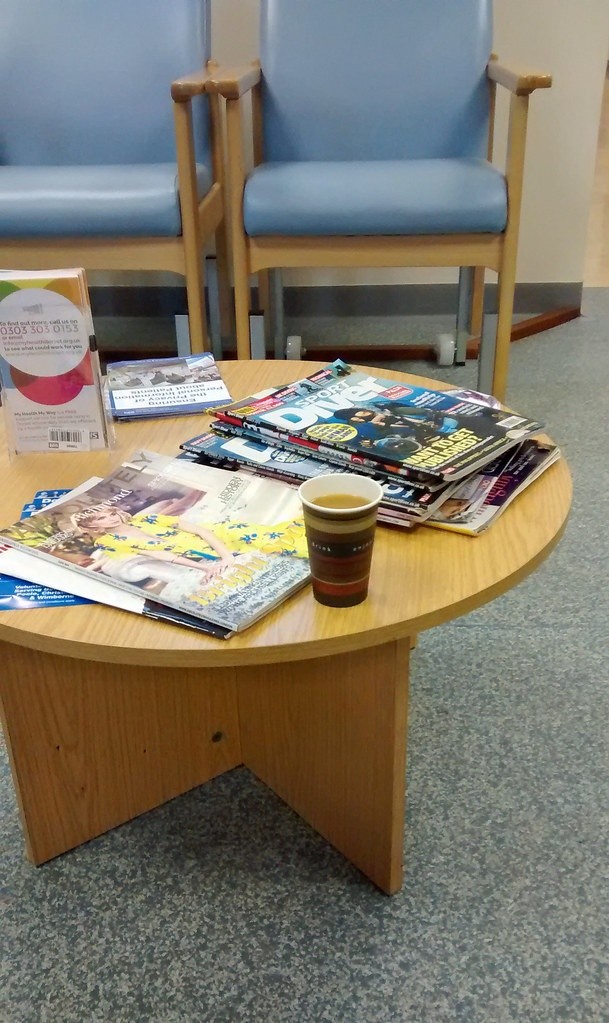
top-left (0, 444), bottom-right (311, 639)
top-left (106, 352), bottom-right (233, 423)
top-left (0, 267), bottom-right (112, 451)
top-left (173, 357), bottom-right (561, 539)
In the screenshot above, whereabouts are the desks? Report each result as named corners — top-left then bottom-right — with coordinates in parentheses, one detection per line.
top-left (0, 358), bottom-right (572, 894)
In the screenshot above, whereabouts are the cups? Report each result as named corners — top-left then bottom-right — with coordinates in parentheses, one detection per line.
top-left (298, 472), bottom-right (383, 608)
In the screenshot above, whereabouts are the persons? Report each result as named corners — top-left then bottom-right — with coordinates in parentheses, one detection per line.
top-left (334, 408), bottom-right (429, 433)
top-left (71, 503), bottom-right (309, 585)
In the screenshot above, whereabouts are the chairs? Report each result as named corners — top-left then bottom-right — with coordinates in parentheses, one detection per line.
top-left (0, 0), bottom-right (233, 363)
top-left (204, 0), bottom-right (552, 407)
